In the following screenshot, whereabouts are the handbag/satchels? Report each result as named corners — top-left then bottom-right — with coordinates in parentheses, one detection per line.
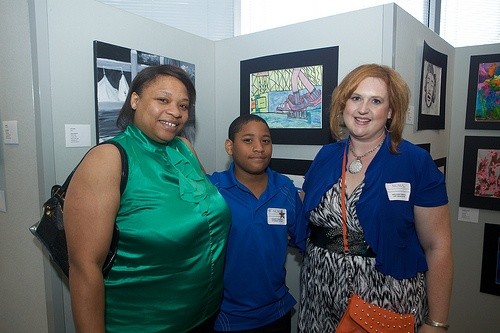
top-left (335, 293), bottom-right (414, 333)
top-left (29, 140), bottom-right (129, 279)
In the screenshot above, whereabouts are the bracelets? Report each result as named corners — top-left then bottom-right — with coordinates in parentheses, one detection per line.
top-left (429, 320), bottom-right (448, 328)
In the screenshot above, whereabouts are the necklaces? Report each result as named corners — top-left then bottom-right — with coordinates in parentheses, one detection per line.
top-left (349, 140), bottom-right (383, 174)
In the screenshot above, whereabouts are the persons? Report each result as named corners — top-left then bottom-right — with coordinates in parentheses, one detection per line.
top-left (302, 63), bottom-right (454, 333)
top-left (206, 114), bottom-right (310, 333)
top-left (64, 64), bottom-right (232, 333)
